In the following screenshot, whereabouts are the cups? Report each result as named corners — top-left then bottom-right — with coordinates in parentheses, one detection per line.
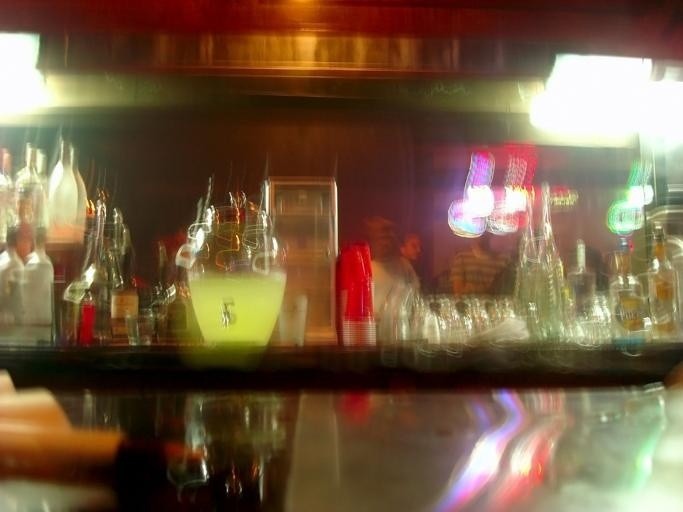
top-left (336, 239), bottom-right (515, 343)
top-left (276, 290), bottom-right (310, 349)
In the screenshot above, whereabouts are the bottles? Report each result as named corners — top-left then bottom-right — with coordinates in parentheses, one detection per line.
top-left (514, 177), bottom-right (568, 343)
top-left (646, 224), bottom-right (680, 336)
top-left (0, 129), bottom-right (190, 347)
top-left (606, 238), bottom-right (648, 338)
top-left (564, 240), bottom-right (605, 340)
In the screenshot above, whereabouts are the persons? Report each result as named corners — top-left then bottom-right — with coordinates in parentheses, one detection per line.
top-left (0, 223), bottom-right (38, 324)
top-left (371, 223), bottom-right (419, 432)
top-left (448, 229), bottom-right (503, 295)
